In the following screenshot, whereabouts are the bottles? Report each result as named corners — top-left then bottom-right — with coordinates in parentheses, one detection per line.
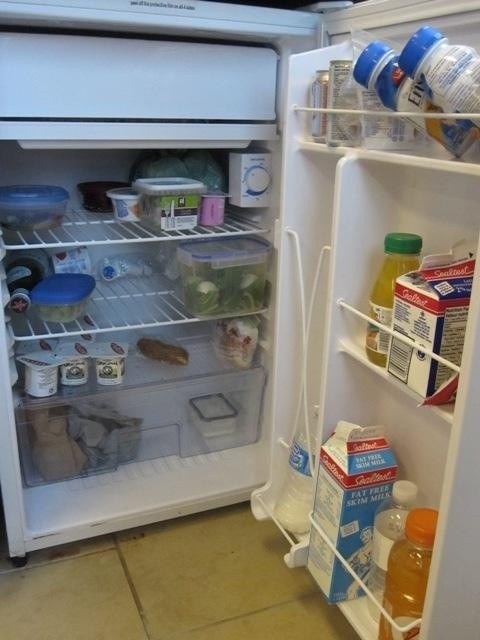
top-left (396, 24), bottom-right (479, 129)
top-left (272, 392), bottom-right (322, 534)
top-left (377, 506), bottom-right (440, 640)
top-left (352, 41), bottom-right (479, 164)
top-left (366, 477), bottom-right (422, 626)
top-left (364, 230), bottom-right (424, 369)
top-left (5, 249), bottom-right (50, 319)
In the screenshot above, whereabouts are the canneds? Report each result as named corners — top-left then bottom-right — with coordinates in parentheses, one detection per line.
top-left (309, 60), bottom-right (420, 147)
